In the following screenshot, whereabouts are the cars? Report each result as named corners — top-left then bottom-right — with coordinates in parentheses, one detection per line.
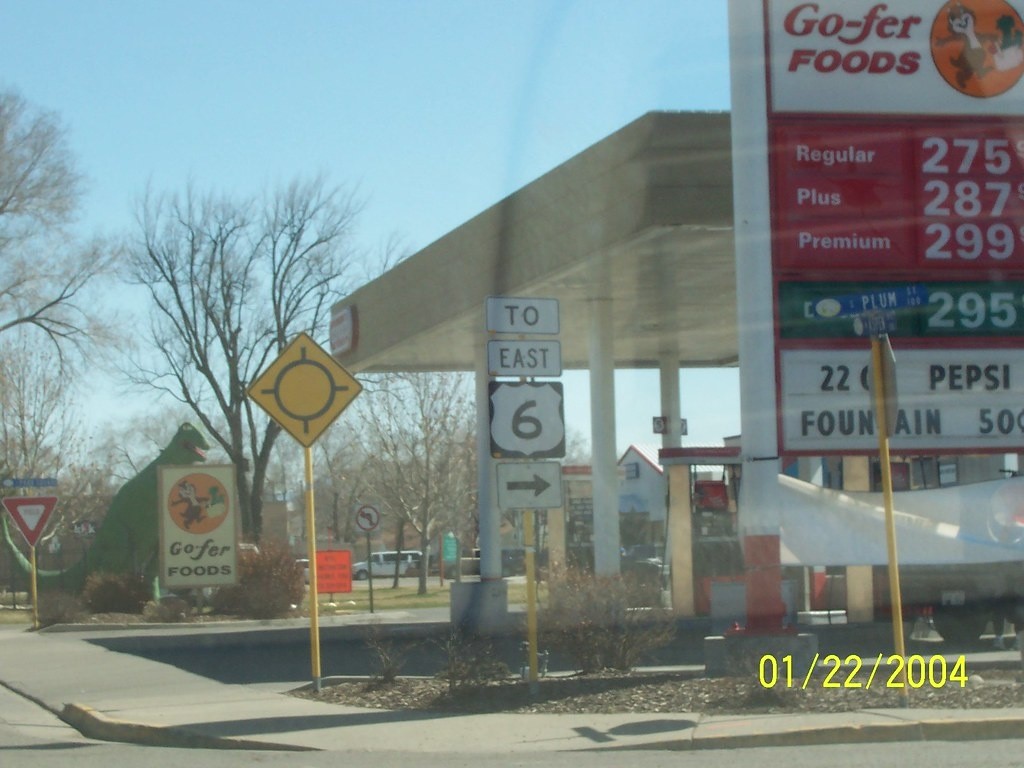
top-left (351, 550), bottom-right (422, 580)
top-left (184, 544), bottom-right (258, 608)
top-left (292, 560), bottom-right (310, 583)
